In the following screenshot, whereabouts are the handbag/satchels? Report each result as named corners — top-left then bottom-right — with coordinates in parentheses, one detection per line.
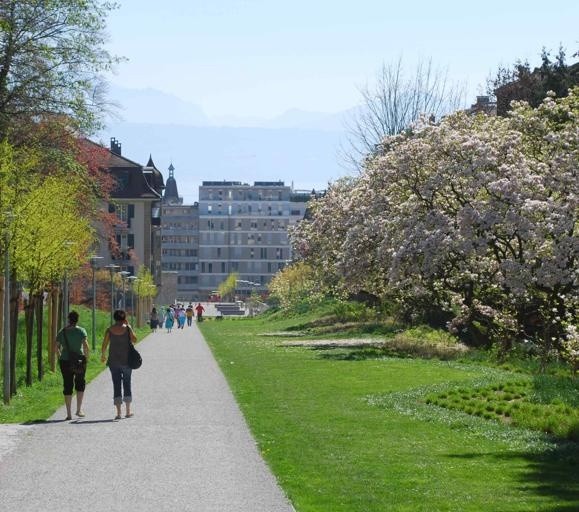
top-left (129, 345), bottom-right (142, 368)
top-left (68, 351), bottom-right (86, 374)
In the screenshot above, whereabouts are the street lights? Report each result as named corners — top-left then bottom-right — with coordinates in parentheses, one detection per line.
top-left (84, 256), bottom-right (104, 351)
top-left (59, 242), bottom-right (75, 329)
top-left (104, 263), bottom-right (157, 330)
top-left (1, 212), bottom-right (15, 391)
top-left (234, 279), bottom-right (261, 318)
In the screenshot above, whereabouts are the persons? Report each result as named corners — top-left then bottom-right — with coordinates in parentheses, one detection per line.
top-left (149, 301), bottom-right (194, 333)
top-left (195, 302), bottom-right (206, 322)
top-left (100, 310), bottom-right (137, 423)
top-left (54, 310), bottom-right (91, 423)
